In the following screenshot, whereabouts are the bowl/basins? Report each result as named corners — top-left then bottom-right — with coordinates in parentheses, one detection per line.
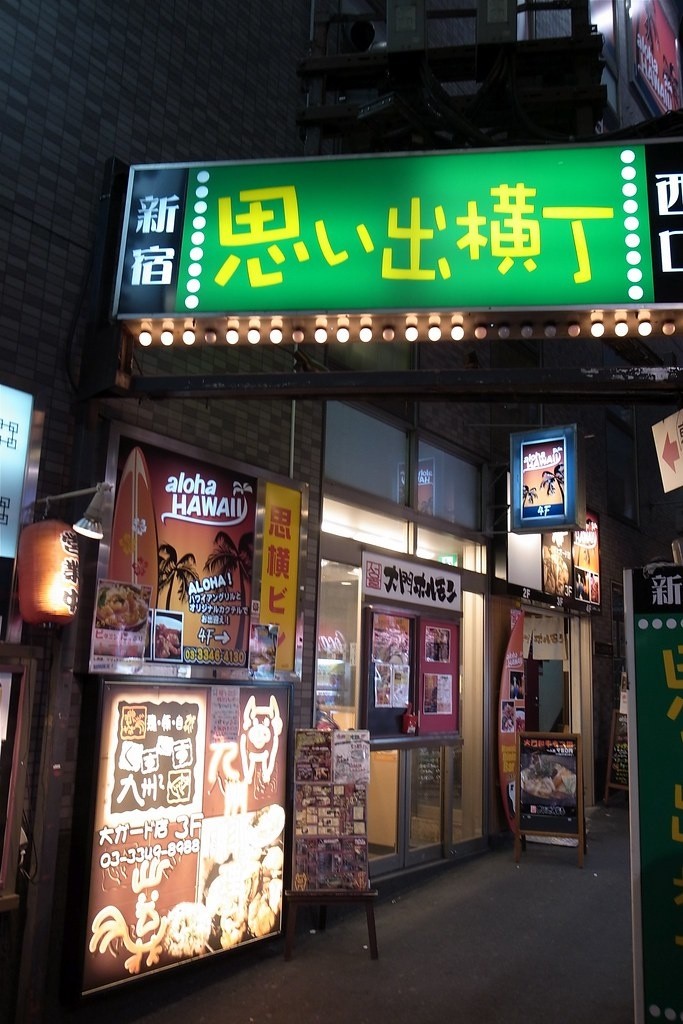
top-left (96, 584), bottom-right (148, 632)
top-left (156, 615), bottom-right (182, 632)
top-left (520, 761), bottom-right (576, 805)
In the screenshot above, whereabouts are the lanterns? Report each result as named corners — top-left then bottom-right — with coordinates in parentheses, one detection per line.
top-left (14, 520), bottom-right (85, 626)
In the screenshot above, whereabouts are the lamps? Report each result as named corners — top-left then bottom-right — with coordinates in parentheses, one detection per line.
top-left (37, 480), bottom-right (114, 540)
